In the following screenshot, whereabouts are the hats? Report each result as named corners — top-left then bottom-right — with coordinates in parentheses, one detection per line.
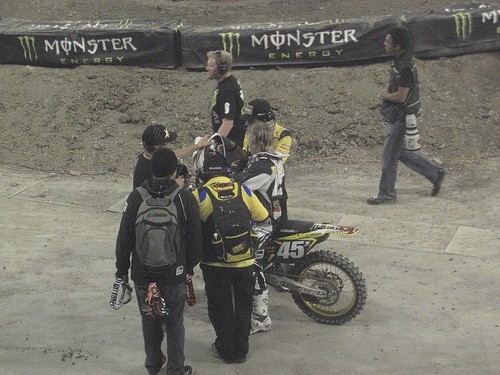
top-left (203, 154), bottom-right (229, 180)
top-left (142, 124), bottom-right (178, 146)
top-left (151, 147), bottom-right (177, 177)
top-left (238, 98), bottom-right (273, 122)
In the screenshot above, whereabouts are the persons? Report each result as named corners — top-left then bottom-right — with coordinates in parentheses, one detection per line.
top-left (366, 27), bottom-right (445, 204)
top-left (205, 50), bottom-right (244, 149)
top-left (233, 123), bottom-right (286, 336)
top-left (133, 124), bottom-right (177, 332)
top-left (193, 154), bottom-right (268, 363)
top-left (109, 148), bottom-right (203, 375)
top-left (244, 99), bottom-right (292, 220)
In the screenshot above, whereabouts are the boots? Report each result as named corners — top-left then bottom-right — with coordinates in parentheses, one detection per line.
top-left (250, 288), bottom-right (272, 334)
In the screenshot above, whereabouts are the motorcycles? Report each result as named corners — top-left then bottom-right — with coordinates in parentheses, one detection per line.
top-left (187, 141), bottom-right (367, 326)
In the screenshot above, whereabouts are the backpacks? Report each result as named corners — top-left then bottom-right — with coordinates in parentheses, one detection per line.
top-left (134, 186), bottom-right (181, 272)
top-left (203, 182), bottom-right (253, 263)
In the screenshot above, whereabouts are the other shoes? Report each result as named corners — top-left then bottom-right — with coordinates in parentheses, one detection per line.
top-left (367, 196), bottom-right (396, 205)
top-left (432, 167), bottom-right (446, 196)
top-left (160, 355), bottom-right (167, 370)
top-left (180, 365), bottom-right (192, 375)
top-left (211, 344), bottom-right (228, 365)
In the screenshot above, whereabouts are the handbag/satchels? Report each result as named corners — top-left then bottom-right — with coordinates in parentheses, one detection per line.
top-left (379, 100), bottom-right (401, 122)
